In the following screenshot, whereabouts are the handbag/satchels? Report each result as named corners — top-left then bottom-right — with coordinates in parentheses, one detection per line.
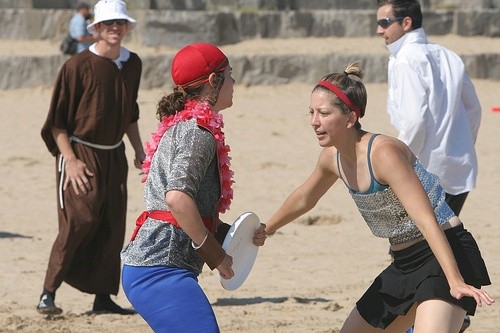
top-left (60, 37), bottom-right (78, 56)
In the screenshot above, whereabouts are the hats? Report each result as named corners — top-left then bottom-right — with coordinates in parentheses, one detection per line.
top-left (172, 42), bottom-right (230, 90)
top-left (86, 0), bottom-right (137, 34)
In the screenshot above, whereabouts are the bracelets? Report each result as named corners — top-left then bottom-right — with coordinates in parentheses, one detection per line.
top-left (266, 230), bottom-right (276, 235)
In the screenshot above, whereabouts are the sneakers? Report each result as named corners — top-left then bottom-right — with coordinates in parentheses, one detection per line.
top-left (36, 295), bottom-right (62, 315)
top-left (459, 316), bottom-right (472, 333)
top-left (93, 299), bottom-right (135, 316)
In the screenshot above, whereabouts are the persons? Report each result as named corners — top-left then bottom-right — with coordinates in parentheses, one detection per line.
top-left (120, 42), bottom-right (267, 333)
top-left (376, 0), bottom-right (482, 333)
top-left (36, 0), bottom-right (146, 314)
top-left (68, 3), bottom-right (98, 53)
top-left (265, 61), bottom-right (496, 333)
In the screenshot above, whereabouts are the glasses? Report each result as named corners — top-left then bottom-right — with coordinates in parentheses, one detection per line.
top-left (375, 18), bottom-right (404, 29)
top-left (101, 19), bottom-right (130, 25)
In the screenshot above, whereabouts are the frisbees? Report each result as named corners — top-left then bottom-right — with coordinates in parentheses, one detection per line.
top-left (220, 212), bottom-right (262, 291)
